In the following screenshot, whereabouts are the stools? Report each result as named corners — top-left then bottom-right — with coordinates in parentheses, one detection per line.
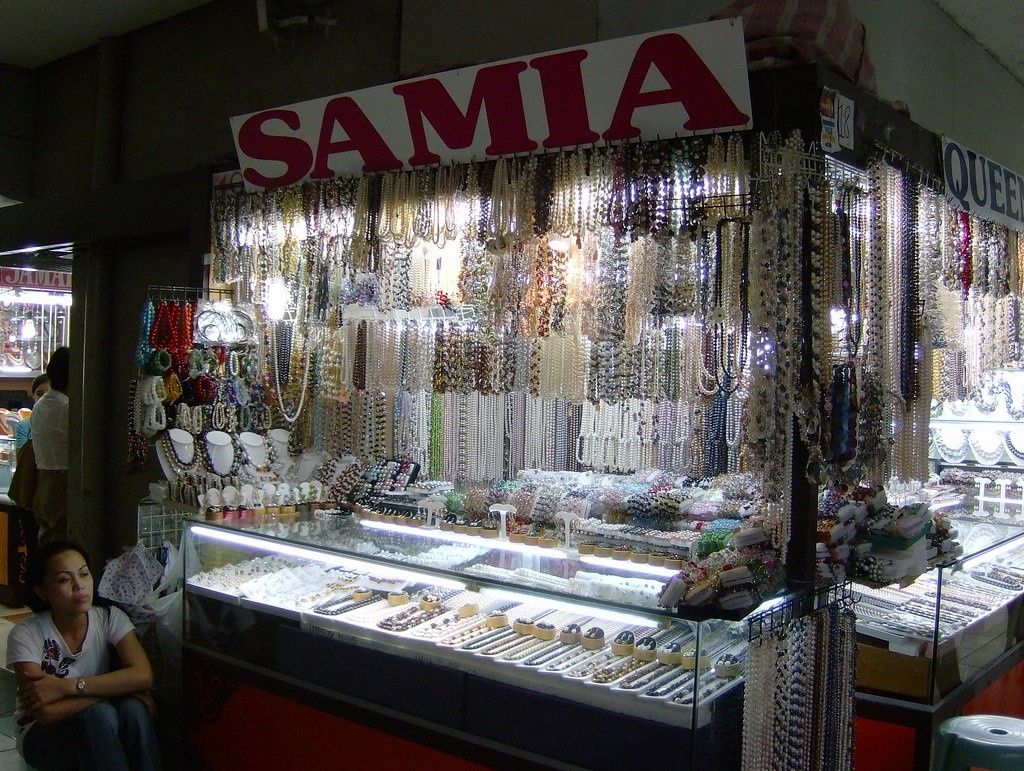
top-left (930, 714), bottom-right (1024, 771)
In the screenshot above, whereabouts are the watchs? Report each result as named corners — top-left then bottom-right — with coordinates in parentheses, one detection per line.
top-left (77, 678), bottom-right (86, 697)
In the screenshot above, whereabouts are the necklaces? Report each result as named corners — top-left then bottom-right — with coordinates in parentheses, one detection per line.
top-left (741, 604), bottom-right (858, 771)
top-left (0, 301), bottom-right (66, 369)
top-left (126, 129), bottom-right (1024, 703)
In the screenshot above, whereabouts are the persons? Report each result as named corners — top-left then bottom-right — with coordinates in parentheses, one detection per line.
top-left (6, 540), bottom-right (161, 771)
top-left (15, 346), bottom-right (70, 613)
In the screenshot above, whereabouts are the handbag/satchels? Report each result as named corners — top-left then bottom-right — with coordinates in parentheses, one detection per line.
top-left (120, 530), bottom-right (190, 704)
top-left (8, 439), bottom-right (35, 511)
